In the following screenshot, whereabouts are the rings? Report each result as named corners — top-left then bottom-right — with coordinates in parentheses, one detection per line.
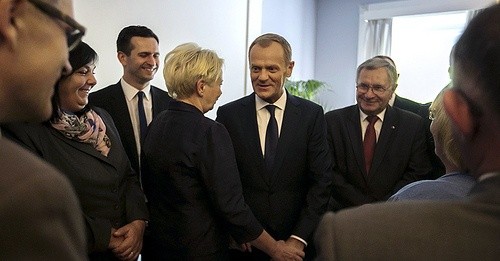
top-left (131, 252), bottom-right (135, 255)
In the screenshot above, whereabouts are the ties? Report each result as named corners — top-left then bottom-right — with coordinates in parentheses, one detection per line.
top-left (136, 90), bottom-right (148, 168)
top-left (363, 115), bottom-right (380, 176)
top-left (263, 105), bottom-right (279, 168)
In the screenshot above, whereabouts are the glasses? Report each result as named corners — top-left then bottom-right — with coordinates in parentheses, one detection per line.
top-left (28, 0), bottom-right (85, 50)
top-left (356, 83), bottom-right (390, 94)
top-left (429, 112), bottom-right (436, 121)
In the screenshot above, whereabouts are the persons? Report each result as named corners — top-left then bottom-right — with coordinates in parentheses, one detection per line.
top-left (325, 59), bottom-right (432, 211)
top-left (0, 0), bottom-right (500, 261)
